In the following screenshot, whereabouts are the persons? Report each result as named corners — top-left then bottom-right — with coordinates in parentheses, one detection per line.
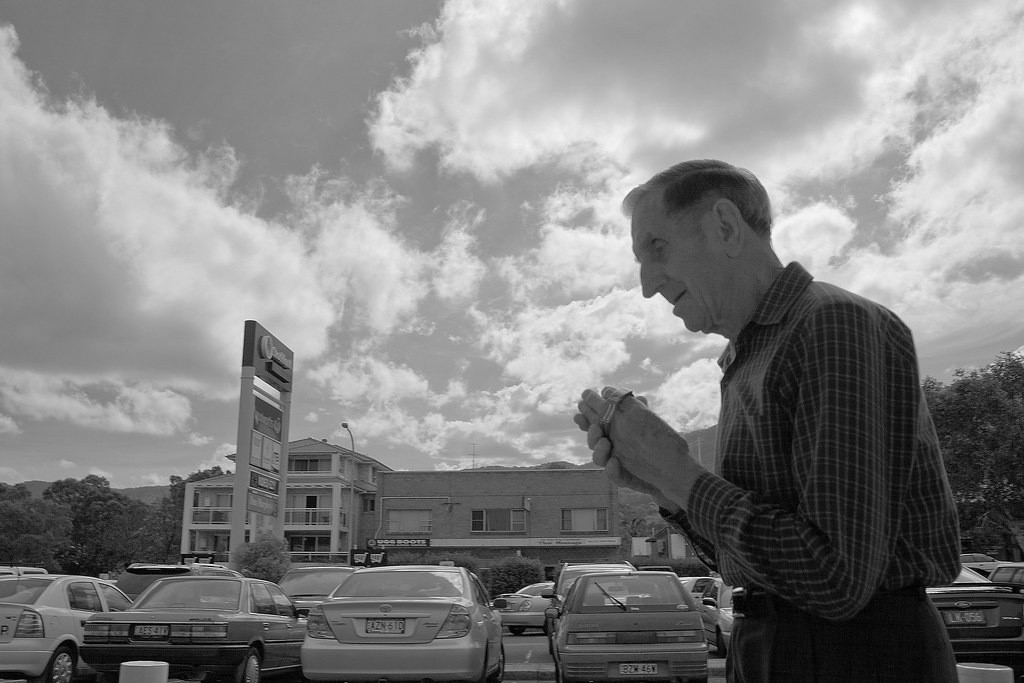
top-left (574, 158), bottom-right (963, 683)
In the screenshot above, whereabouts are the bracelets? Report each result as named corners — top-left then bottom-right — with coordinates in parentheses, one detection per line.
top-left (599, 389), bottom-right (632, 437)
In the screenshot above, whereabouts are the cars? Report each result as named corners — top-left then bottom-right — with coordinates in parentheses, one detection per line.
top-left (0, 553), bottom-right (1024, 683)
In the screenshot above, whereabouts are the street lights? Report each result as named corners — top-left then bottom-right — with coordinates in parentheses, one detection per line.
top-left (342, 422), bottom-right (354, 567)
